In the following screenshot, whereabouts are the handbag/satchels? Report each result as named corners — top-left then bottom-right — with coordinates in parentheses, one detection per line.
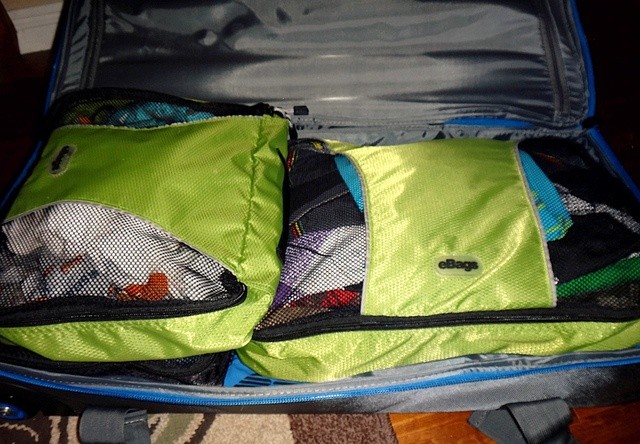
top-left (0, 0), bottom-right (639, 412)
